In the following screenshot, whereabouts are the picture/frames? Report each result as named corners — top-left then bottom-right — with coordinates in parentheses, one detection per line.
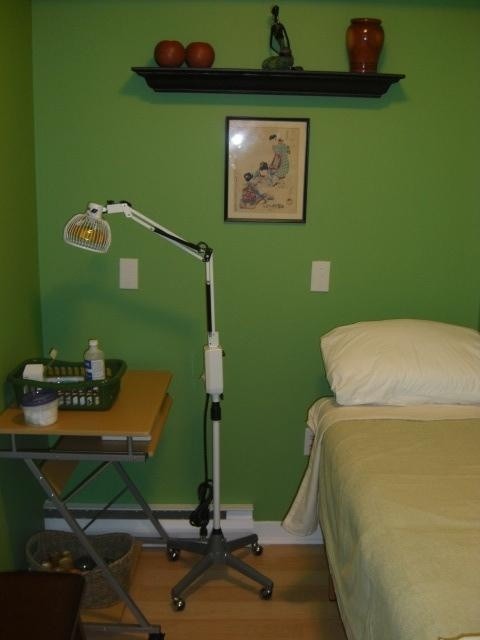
top-left (224, 115), bottom-right (309, 223)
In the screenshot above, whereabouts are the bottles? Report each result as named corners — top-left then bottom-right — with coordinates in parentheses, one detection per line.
top-left (83, 339), bottom-right (107, 384)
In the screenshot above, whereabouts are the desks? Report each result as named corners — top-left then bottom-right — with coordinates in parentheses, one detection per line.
top-left (0, 370), bottom-right (179, 639)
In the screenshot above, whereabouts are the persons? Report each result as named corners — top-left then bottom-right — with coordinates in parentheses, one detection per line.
top-left (262, 5), bottom-right (302, 71)
top-left (239, 134), bottom-right (292, 209)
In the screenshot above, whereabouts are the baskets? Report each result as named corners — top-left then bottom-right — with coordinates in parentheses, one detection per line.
top-left (6, 358), bottom-right (127, 411)
top-left (25, 530), bottom-right (136, 609)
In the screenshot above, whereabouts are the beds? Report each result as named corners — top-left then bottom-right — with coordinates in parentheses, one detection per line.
top-left (314, 396), bottom-right (479, 640)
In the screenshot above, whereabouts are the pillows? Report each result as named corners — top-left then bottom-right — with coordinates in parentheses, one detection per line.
top-left (321, 318), bottom-right (480, 405)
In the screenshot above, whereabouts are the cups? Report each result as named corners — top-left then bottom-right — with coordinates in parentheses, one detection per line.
top-left (20, 390), bottom-right (60, 427)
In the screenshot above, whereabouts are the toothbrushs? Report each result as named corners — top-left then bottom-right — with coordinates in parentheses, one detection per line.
top-left (43, 346), bottom-right (60, 370)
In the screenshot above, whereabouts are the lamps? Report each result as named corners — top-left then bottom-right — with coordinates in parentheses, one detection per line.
top-left (63, 200), bottom-right (273, 610)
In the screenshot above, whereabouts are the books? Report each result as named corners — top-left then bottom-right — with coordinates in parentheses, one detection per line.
top-left (102, 437), bottom-right (151, 441)
